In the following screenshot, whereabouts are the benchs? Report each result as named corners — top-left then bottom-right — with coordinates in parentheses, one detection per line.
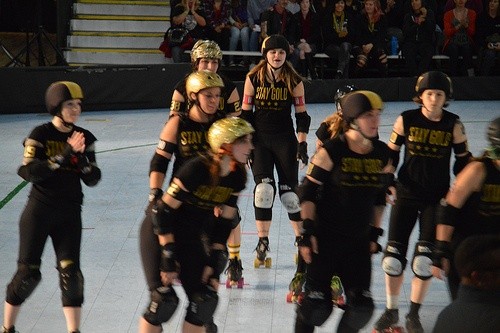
top-left (185, 50), bottom-right (399, 59)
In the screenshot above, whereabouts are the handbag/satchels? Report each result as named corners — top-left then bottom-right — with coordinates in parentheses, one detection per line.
top-left (168, 27), bottom-right (188, 41)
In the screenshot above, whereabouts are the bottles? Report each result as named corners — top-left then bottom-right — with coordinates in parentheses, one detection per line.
top-left (392, 37), bottom-right (398, 54)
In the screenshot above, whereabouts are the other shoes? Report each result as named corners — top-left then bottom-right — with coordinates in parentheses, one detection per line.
top-left (238, 60), bottom-right (249, 68)
top-left (357, 54), bottom-right (368, 67)
top-left (337, 64), bottom-right (345, 75)
top-left (227, 60), bottom-right (236, 66)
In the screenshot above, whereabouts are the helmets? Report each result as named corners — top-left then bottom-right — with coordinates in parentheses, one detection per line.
top-left (185, 69), bottom-right (224, 100)
top-left (342, 91), bottom-right (383, 121)
top-left (483, 117), bottom-right (500, 145)
top-left (190, 39), bottom-right (222, 62)
top-left (45, 81), bottom-right (83, 114)
top-left (261, 34), bottom-right (291, 58)
top-left (208, 116), bottom-right (254, 154)
top-left (415, 72), bottom-right (454, 95)
top-left (334, 83), bottom-right (358, 101)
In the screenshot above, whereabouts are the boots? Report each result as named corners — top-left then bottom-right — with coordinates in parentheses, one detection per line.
top-left (304, 52), bottom-right (318, 80)
top-left (299, 59), bottom-right (312, 81)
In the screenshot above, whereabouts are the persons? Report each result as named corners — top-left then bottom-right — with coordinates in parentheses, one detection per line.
top-left (228, 0), bottom-right (250, 64)
top-left (286, 84), bottom-right (362, 305)
top-left (171, 0), bottom-right (208, 63)
top-left (147, 69), bottom-right (244, 289)
top-left (324, 0), bottom-right (351, 79)
top-left (430, 116), bottom-right (499, 333)
top-left (441, 0), bottom-right (500, 77)
top-left (373, 71), bottom-right (472, 333)
top-left (202, 0), bottom-right (232, 66)
top-left (241, 34), bottom-right (311, 267)
top-left (291, 0), bottom-right (322, 80)
top-left (294, 91), bottom-right (394, 333)
top-left (259, 0), bottom-right (293, 36)
top-left (247, 0), bottom-right (276, 30)
top-left (169, 39), bottom-right (242, 117)
top-left (0, 81), bottom-right (101, 333)
top-left (139, 116), bottom-right (255, 333)
top-left (286, 0), bottom-right (300, 14)
top-left (345, 0), bottom-right (436, 78)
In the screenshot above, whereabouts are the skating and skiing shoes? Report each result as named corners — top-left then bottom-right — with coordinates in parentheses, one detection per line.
top-left (285, 271), bottom-right (307, 303)
top-left (224, 256), bottom-right (244, 289)
top-left (403, 313), bottom-right (424, 333)
top-left (252, 236), bottom-right (272, 269)
top-left (331, 275), bottom-right (345, 307)
top-left (371, 308), bottom-right (405, 333)
